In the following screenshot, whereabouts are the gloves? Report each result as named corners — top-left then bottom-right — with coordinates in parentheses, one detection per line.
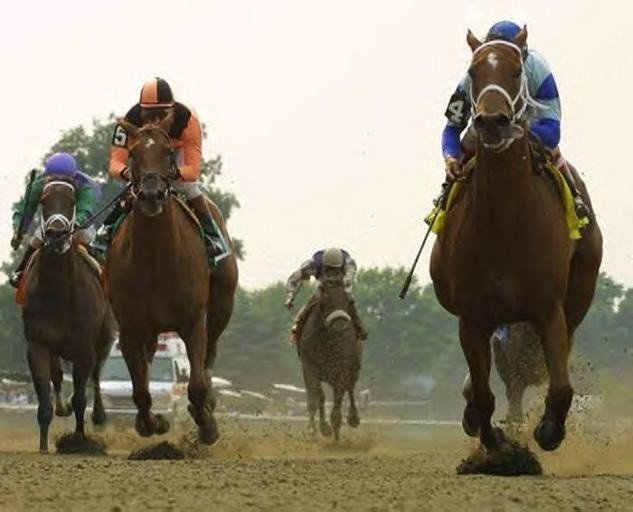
top-left (443, 154), bottom-right (463, 183)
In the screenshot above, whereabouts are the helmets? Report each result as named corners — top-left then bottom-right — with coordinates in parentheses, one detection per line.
top-left (45, 150), bottom-right (78, 178)
top-left (138, 77), bottom-right (174, 108)
top-left (322, 247), bottom-right (343, 268)
top-left (484, 21), bottom-right (530, 57)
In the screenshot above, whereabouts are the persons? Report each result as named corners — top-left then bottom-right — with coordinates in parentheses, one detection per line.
top-left (420, 21), bottom-right (592, 243)
top-left (6, 151), bottom-right (104, 289)
top-left (281, 248), bottom-right (368, 348)
top-left (88, 74), bottom-right (230, 268)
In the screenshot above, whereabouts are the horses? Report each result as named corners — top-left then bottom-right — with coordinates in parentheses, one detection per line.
top-left (102, 110), bottom-right (240, 447)
top-left (295, 263), bottom-right (361, 443)
top-left (429, 22), bottom-right (603, 451)
top-left (18, 174), bottom-right (115, 455)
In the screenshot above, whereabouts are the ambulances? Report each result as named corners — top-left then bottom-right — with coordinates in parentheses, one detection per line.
top-left (83, 331), bottom-right (192, 416)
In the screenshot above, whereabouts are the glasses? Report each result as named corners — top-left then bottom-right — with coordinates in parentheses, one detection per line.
top-left (142, 112), bottom-right (166, 123)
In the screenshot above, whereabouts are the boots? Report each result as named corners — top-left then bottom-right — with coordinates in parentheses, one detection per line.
top-left (88, 201), bottom-right (123, 252)
top-left (434, 147), bottom-right (472, 210)
top-left (559, 163), bottom-right (589, 219)
top-left (349, 300), bottom-right (365, 338)
top-left (188, 193), bottom-right (225, 257)
top-left (9, 244), bottom-right (37, 289)
top-left (292, 304), bottom-right (310, 334)
